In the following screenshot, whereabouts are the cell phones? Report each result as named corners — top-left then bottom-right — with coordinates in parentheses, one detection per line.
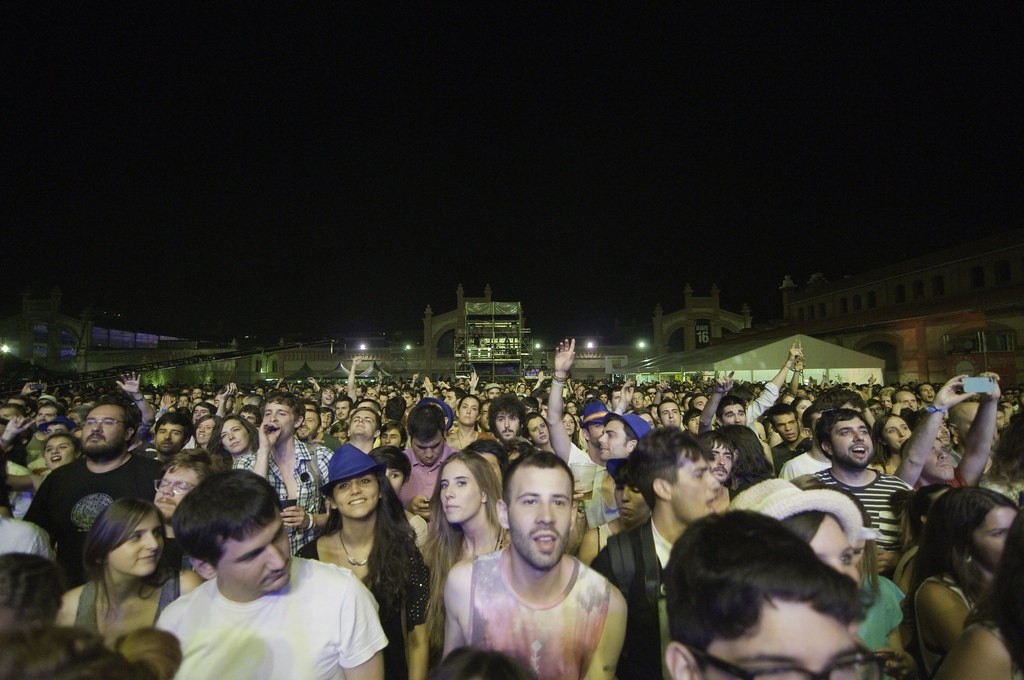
top-left (961, 376), bottom-right (994, 393)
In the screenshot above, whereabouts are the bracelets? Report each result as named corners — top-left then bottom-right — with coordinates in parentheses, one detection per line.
top-left (305, 513), bottom-right (313, 530)
top-left (133, 396), bottom-right (144, 402)
top-left (552, 371), bottom-right (569, 382)
top-left (552, 380), bottom-right (565, 387)
top-left (927, 405), bottom-right (949, 415)
top-left (784, 365), bottom-right (791, 369)
top-left (41, 391), bottom-right (46, 393)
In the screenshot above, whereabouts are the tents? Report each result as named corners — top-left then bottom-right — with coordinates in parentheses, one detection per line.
top-left (611, 333), bottom-right (887, 385)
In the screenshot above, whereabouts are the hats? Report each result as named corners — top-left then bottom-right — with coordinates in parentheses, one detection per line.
top-left (318, 443), bottom-right (386, 499)
top-left (603, 410), bottom-right (652, 443)
top-left (37, 415), bottom-right (77, 433)
top-left (484, 383), bottom-right (502, 391)
top-left (606, 458), bottom-right (630, 475)
top-left (580, 401), bottom-right (611, 428)
top-left (37, 394), bottom-right (57, 405)
top-left (725, 473), bottom-right (880, 549)
top-left (416, 397), bottom-right (454, 432)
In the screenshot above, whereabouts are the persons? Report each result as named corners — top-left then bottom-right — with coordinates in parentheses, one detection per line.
top-left (0, 339), bottom-right (1024, 680)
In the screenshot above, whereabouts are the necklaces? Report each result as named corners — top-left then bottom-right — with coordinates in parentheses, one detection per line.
top-left (339, 529), bottom-right (368, 565)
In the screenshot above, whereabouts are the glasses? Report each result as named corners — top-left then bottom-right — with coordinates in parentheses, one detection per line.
top-left (648, 392), bottom-right (657, 395)
top-left (683, 642), bottom-right (895, 680)
top-left (154, 479), bottom-right (197, 495)
top-left (84, 417), bottom-right (128, 427)
